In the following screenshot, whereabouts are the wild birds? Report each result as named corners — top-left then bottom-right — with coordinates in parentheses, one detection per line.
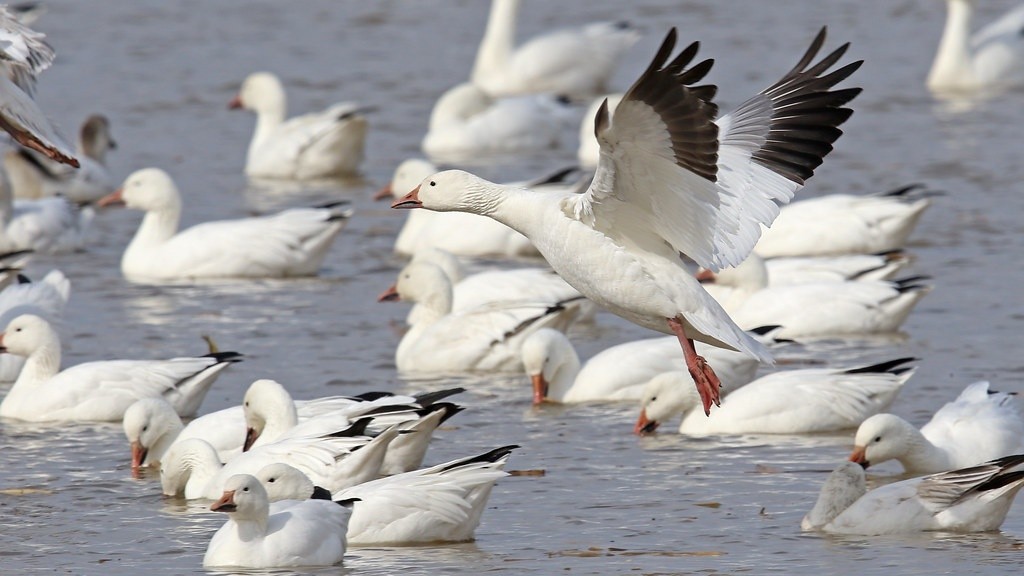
top-left (96, 168), bottom-right (355, 286)
top-left (372, 1), bottom-right (1024, 535)
top-left (0, 313), bottom-right (245, 424)
top-left (226, 70), bottom-right (375, 181)
top-left (123, 378), bottom-right (522, 569)
top-left (0, 0), bottom-right (119, 377)
